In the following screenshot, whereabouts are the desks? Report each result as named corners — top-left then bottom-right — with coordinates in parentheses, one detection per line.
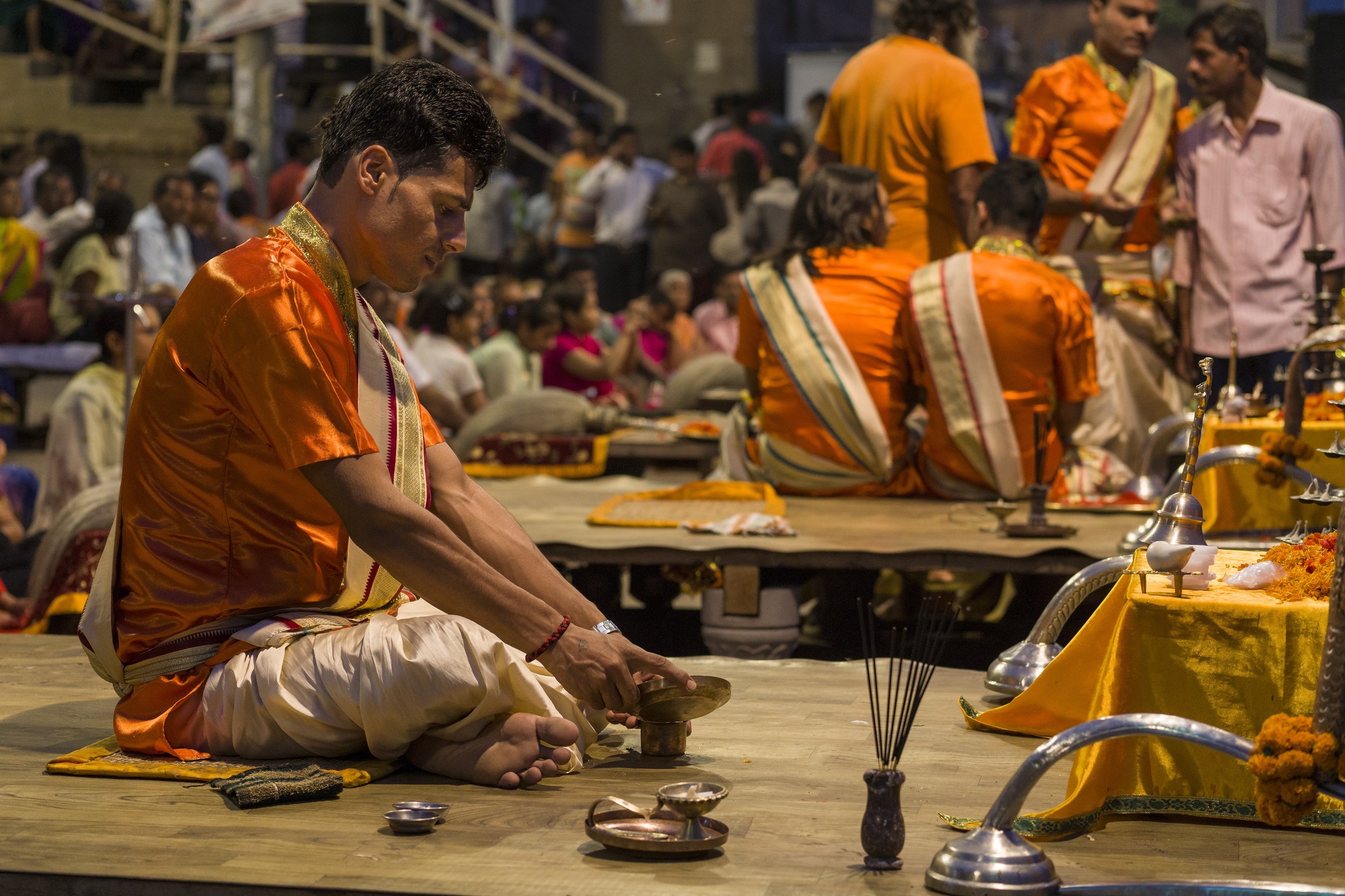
top-left (1196, 414), bottom-right (1345, 537)
top-left (934, 543), bottom-right (1344, 838)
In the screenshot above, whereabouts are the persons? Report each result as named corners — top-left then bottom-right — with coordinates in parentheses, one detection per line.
top-left (0, 0), bottom-right (1345, 631)
top-left (78, 54), bottom-right (697, 788)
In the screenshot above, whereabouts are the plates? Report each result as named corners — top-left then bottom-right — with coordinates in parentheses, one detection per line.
top-left (634, 674), bottom-right (732, 720)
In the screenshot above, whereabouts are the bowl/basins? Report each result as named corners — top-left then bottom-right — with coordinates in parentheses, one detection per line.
top-left (382, 801), bottom-right (451, 833)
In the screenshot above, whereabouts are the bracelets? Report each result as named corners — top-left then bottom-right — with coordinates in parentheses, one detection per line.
top-left (589, 620), bottom-right (622, 635)
top-left (526, 616), bottom-right (572, 663)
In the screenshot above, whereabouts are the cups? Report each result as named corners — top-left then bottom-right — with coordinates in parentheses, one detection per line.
top-left (635, 719), bottom-right (688, 756)
